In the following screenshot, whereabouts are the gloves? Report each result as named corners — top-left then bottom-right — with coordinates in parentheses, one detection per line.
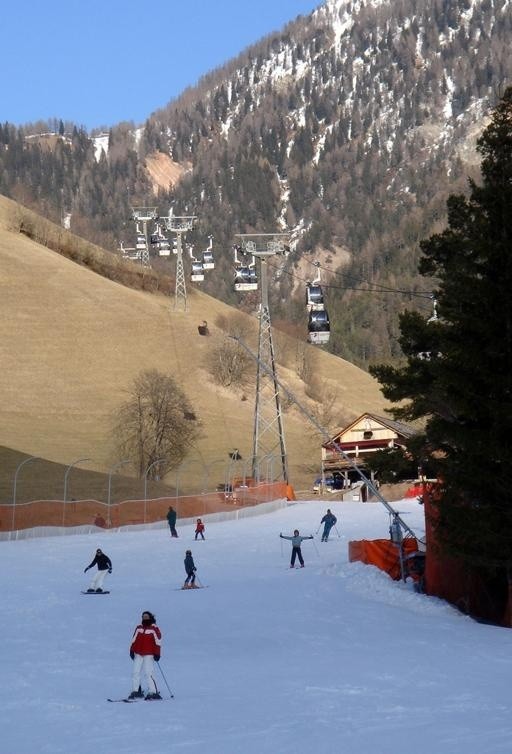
top-left (154, 655), bottom-right (160, 661)
top-left (309, 537), bottom-right (313, 539)
top-left (130, 651), bottom-right (134, 659)
top-left (84, 568), bottom-right (88, 573)
top-left (193, 567), bottom-right (196, 571)
top-left (108, 568), bottom-right (112, 573)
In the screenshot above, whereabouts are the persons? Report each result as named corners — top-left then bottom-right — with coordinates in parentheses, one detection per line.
top-left (182, 549), bottom-right (198, 588)
top-left (320, 508), bottom-right (337, 542)
top-left (194, 518), bottom-right (206, 540)
top-left (84, 548), bottom-right (112, 592)
top-left (128, 611), bottom-right (163, 699)
top-left (95, 512), bottom-right (105, 527)
top-left (280, 529), bottom-right (314, 568)
top-left (166, 506), bottom-right (178, 536)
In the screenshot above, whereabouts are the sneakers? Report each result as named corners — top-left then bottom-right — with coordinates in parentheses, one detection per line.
top-left (86, 589), bottom-right (102, 592)
top-left (321, 539), bottom-right (327, 542)
top-left (290, 563), bottom-right (304, 568)
top-left (128, 691), bottom-right (144, 699)
top-left (145, 694), bottom-right (158, 700)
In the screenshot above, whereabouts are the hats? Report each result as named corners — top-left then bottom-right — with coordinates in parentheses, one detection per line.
top-left (186, 550), bottom-right (191, 556)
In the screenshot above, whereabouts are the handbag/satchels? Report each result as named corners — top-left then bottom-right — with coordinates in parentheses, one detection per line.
top-left (280, 535), bottom-right (283, 537)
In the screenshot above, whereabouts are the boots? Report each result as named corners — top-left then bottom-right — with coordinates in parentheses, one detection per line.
top-left (184, 582), bottom-right (198, 588)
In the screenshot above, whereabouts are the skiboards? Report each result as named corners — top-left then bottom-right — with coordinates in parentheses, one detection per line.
top-left (174, 585), bottom-right (209, 591)
top-left (107, 698), bottom-right (170, 703)
top-left (81, 591), bottom-right (110, 594)
